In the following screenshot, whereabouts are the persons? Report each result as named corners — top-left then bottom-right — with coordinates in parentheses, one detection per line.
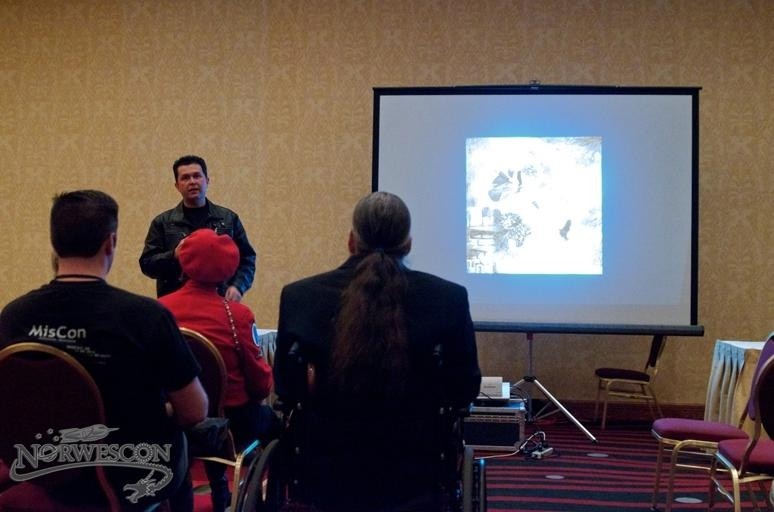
top-left (272, 191), bottom-right (481, 512)
top-left (139, 156), bottom-right (256, 303)
top-left (0, 189), bottom-right (210, 512)
top-left (157, 228), bottom-right (285, 512)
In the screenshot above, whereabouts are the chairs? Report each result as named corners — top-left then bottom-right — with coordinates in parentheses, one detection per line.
top-left (0, 327), bottom-right (238, 511)
top-left (594, 334), bottom-right (774, 512)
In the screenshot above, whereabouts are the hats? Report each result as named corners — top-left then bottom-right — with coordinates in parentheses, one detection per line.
top-left (179, 228), bottom-right (240, 283)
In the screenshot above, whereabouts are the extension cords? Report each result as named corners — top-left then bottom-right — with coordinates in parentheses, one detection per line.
top-left (532, 447), bottom-right (553, 460)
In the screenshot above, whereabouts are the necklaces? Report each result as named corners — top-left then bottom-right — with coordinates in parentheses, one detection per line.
top-left (55, 273), bottom-right (100, 281)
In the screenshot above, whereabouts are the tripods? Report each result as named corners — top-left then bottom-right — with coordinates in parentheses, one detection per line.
top-left (511, 331), bottom-right (599, 444)
